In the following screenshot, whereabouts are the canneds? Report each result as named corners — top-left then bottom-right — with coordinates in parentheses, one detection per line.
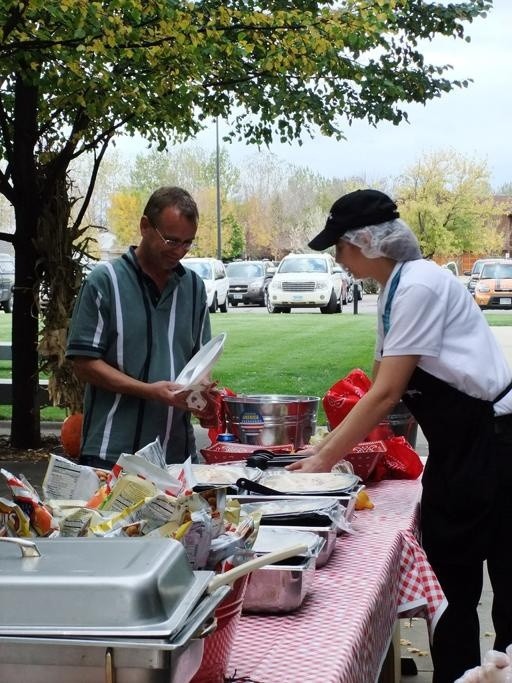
top-left (216, 431), bottom-right (237, 442)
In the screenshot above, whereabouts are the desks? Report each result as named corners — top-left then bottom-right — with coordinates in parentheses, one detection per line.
top-left (219, 455), bottom-right (428, 682)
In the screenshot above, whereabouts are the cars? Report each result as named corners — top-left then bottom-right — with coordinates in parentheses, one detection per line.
top-left (464, 258), bottom-right (488, 298)
top-left (266, 250), bottom-right (364, 315)
top-left (472, 260), bottom-right (510, 311)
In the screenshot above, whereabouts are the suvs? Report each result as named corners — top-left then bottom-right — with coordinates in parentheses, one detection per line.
top-left (0, 252), bottom-right (16, 315)
top-left (181, 257), bottom-right (229, 314)
top-left (226, 257), bottom-right (272, 309)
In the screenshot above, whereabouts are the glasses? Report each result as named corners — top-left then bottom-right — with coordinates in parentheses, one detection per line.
top-left (149, 221), bottom-right (196, 248)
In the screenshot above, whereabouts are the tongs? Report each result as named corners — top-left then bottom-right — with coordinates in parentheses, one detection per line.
top-left (246, 450), bottom-right (308, 470)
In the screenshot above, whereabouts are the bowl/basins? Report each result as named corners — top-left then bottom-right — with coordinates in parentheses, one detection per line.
top-left (323, 387), bottom-right (418, 452)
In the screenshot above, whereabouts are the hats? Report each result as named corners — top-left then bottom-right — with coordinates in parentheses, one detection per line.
top-left (305, 188), bottom-right (400, 253)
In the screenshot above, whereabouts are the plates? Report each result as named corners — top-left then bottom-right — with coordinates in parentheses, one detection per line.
top-left (171, 333), bottom-right (227, 396)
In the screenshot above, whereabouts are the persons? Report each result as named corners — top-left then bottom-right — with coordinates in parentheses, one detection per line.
top-left (64, 186), bottom-right (214, 470)
top-left (285, 189), bottom-right (512, 683)
